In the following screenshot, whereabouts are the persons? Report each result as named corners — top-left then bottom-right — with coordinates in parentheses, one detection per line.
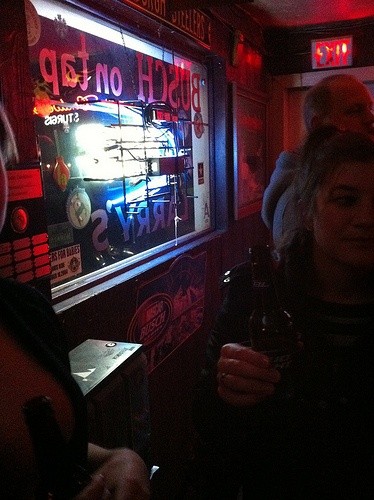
top-left (260, 74), bottom-right (374, 245)
top-left (175, 130), bottom-right (374, 500)
top-left (38, 134), bottom-right (84, 226)
top-left (0, 108), bottom-right (151, 500)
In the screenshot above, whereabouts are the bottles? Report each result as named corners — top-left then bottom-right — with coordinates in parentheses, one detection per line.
top-left (249, 254), bottom-right (314, 448)
top-left (21, 397), bottom-right (91, 500)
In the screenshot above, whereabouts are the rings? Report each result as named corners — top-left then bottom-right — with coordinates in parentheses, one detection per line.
top-left (220, 373), bottom-right (227, 384)
top-left (103, 488), bottom-right (114, 496)
top-left (99, 474), bottom-right (106, 481)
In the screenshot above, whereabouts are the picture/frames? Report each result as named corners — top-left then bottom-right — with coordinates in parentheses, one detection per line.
top-left (228, 80), bottom-right (269, 221)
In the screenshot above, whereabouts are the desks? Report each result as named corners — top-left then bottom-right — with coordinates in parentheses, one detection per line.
top-left (69, 337), bottom-right (154, 469)
